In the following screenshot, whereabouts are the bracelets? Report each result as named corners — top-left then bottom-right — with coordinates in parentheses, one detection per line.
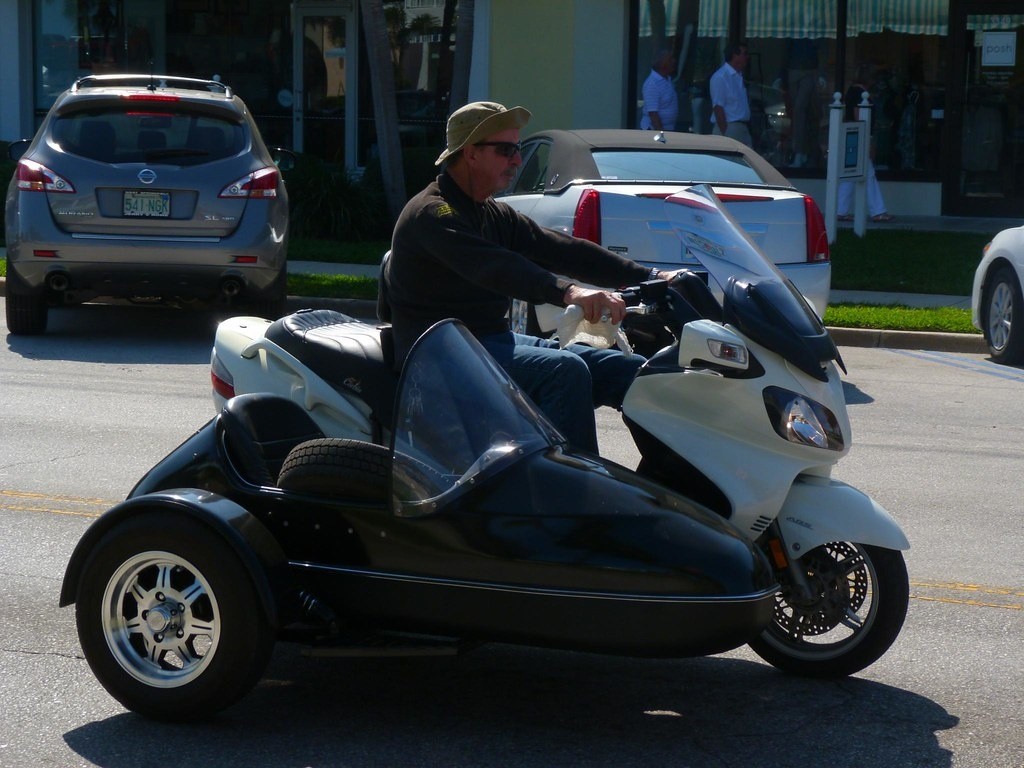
top-left (648, 267), bottom-right (661, 280)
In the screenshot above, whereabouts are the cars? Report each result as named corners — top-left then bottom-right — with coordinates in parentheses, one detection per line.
top-left (5, 75), bottom-right (296, 342)
top-left (970, 225), bottom-right (1024, 365)
top-left (490, 129), bottom-right (834, 326)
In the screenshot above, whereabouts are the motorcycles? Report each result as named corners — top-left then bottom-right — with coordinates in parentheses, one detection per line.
top-left (57, 268), bottom-right (912, 730)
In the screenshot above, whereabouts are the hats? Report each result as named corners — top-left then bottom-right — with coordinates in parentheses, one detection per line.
top-left (434, 102), bottom-right (532, 166)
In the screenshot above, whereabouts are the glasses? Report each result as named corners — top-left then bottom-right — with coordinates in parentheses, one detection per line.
top-left (475, 140), bottom-right (522, 157)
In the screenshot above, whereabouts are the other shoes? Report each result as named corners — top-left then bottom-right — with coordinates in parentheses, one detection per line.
top-left (838, 215), bottom-right (854, 221)
top-left (872, 214), bottom-right (895, 223)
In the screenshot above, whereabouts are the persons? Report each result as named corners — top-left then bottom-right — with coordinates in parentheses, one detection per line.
top-left (710, 42), bottom-right (753, 148)
top-left (385, 100), bottom-right (696, 457)
top-left (639, 50), bottom-right (678, 133)
top-left (764, 38), bottom-right (831, 167)
top-left (837, 59), bottom-right (896, 223)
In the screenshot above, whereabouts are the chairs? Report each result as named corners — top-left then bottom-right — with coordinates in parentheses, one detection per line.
top-left (138, 131), bottom-right (168, 149)
top-left (187, 126), bottom-right (225, 150)
top-left (79, 120), bottom-right (118, 155)
top-left (222, 393), bottom-right (325, 488)
